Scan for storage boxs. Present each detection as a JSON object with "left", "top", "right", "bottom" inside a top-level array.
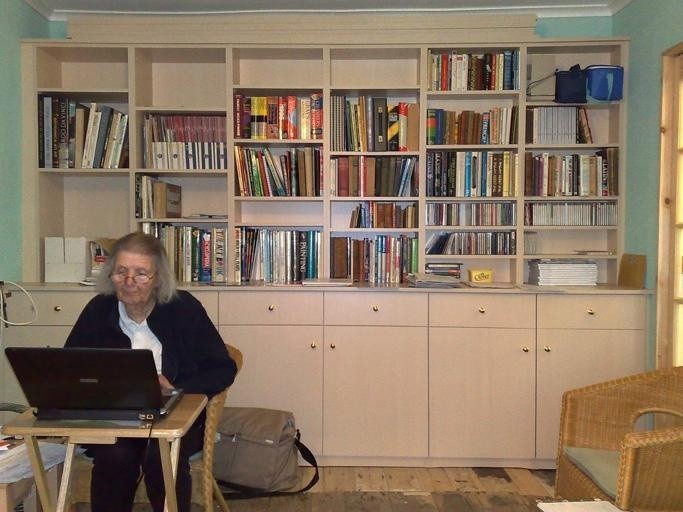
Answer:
[
  {"left": 44, "top": 261, "right": 91, "bottom": 283},
  {"left": 584, "top": 63, "right": 623, "bottom": 103},
  {"left": 552, "top": 63, "right": 588, "bottom": 103},
  {"left": 44, "top": 235, "right": 65, "bottom": 262},
  {"left": 152, "top": 181, "right": 183, "bottom": 218},
  {"left": 64, "top": 237, "right": 93, "bottom": 263}
]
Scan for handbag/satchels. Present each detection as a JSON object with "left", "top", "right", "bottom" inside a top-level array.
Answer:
[
  {"left": 589, "top": 65, "right": 624, "bottom": 101},
  {"left": 555, "top": 65, "right": 588, "bottom": 102}
]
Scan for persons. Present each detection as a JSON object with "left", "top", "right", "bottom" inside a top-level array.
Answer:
[{"left": 63, "top": 229, "right": 238, "bottom": 512}]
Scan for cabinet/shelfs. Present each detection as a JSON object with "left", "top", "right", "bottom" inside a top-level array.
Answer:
[
  {"left": 0, "top": 286, "right": 218, "bottom": 422},
  {"left": 427, "top": 292, "right": 650, "bottom": 470},
  {"left": 216, "top": 289, "right": 429, "bottom": 468},
  {"left": 18, "top": 38, "right": 632, "bottom": 289}
]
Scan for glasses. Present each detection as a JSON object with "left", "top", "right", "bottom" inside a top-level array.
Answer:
[{"left": 109, "top": 272, "right": 151, "bottom": 285}]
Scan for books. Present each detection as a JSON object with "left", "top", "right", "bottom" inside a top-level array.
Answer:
[
  {"left": 350, "top": 203, "right": 418, "bottom": 228},
  {"left": 328, "top": 157, "right": 420, "bottom": 198},
  {"left": 183, "top": 212, "right": 227, "bottom": 219},
  {"left": 425, "top": 230, "right": 515, "bottom": 254},
  {"left": 426, "top": 104, "right": 518, "bottom": 145},
  {"left": 427, "top": 51, "right": 519, "bottom": 91},
  {"left": 524, "top": 230, "right": 538, "bottom": 255},
  {"left": 141, "top": 112, "right": 227, "bottom": 169},
  {"left": 330, "top": 95, "right": 418, "bottom": 151},
  {"left": 525, "top": 107, "right": 596, "bottom": 145},
  {"left": 233, "top": 92, "right": 324, "bottom": 140},
  {"left": 234, "top": 227, "right": 324, "bottom": 285},
  {"left": 425, "top": 202, "right": 517, "bottom": 226},
  {"left": 38, "top": 92, "right": 129, "bottom": 168},
  {"left": 578, "top": 250, "right": 610, "bottom": 256},
  {"left": 524, "top": 149, "right": 619, "bottom": 196},
  {"left": 530, "top": 260, "right": 597, "bottom": 285},
  {"left": 330, "top": 234, "right": 416, "bottom": 283},
  {"left": 426, "top": 149, "right": 518, "bottom": 197},
  {"left": 141, "top": 221, "right": 227, "bottom": 285},
  {"left": 405, "top": 273, "right": 461, "bottom": 289},
  {"left": 464, "top": 282, "right": 514, "bottom": 289},
  {"left": 132, "top": 171, "right": 178, "bottom": 216},
  {"left": 425, "top": 261, "right": 462, "bottom": 287},
  {"left": 524, "top": 202, "right": 618, "bottom": 227},
  {"left": 233, "top": 144, "right": 324, "bottom": 197}
]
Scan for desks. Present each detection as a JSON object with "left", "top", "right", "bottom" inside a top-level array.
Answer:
[{"left": 0, "top": 391, "right": 208, "bottom": 512}]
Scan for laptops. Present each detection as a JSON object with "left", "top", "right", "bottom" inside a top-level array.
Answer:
[{"left": 4, "top": 346, "right": 184, "bottom": 423}]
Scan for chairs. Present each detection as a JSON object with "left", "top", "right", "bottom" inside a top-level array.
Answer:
[
  {"left": 55, "top": 341, "right": 244, "bottom": 512},
  {"left": 553, "top": 365, "right": 682, "bottom": 512}
]
